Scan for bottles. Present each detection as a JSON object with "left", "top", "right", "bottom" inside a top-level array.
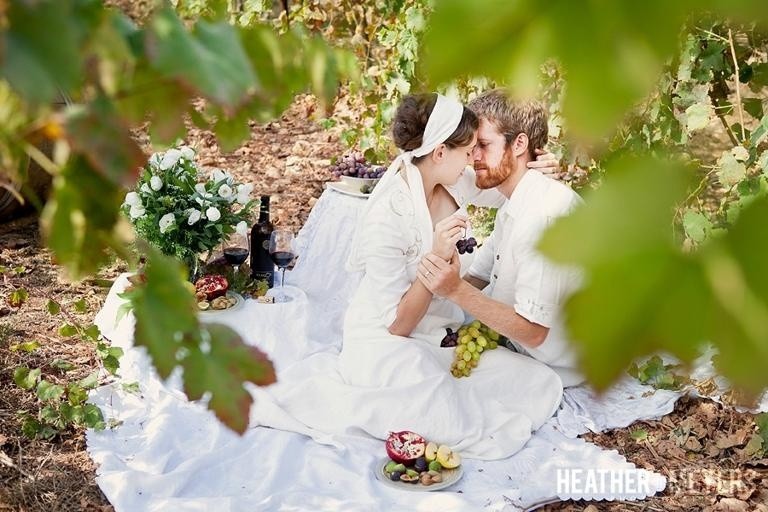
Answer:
[{"left": 250, "top": 195, "right": 273, "bottom": 290}]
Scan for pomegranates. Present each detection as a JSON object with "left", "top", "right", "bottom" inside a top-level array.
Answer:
[
  {"left": 386, "top": 431, "right": 426, "bottom": 466},
  {"left": 196, "top": 274, "right": 229, "bottom": 301}
]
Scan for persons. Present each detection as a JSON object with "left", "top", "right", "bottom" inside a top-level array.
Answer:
[
  {"left": 245, "top": 90, "right": 564, "bottom": 460},
  {"left": 414, "top": 86, "right": 587, "bottom": 388}
]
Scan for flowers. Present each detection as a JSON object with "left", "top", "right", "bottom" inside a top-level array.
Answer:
[{"left": 125, "top": 149, "right": 270, "bottom": 262}]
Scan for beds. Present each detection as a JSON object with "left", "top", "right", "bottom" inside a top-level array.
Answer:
[{"left": 276, "top": 189, "right": 367, "bottom": 285}]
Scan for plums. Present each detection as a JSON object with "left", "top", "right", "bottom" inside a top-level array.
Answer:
[
  {"left": 414, "top": 457, "right": 428, "bottom": 473},
  {"left": 428, "top": 460, "right": 442, "bottom": 472}
]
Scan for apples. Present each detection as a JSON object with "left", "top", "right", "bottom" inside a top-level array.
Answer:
[{"left": 425, "top": 442, "right": 461, "bottom": 469}]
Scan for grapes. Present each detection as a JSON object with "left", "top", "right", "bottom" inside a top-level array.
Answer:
[
  {"left": 328, "top": 155, "right": 388, "bottom": 193},
  {"left": 440, "top": 319, "right": 499, "bottom": 378},
  {"left": 456, "top": 237, "right": 477, "bottom": 254},
  {"left": 391, "top": 471, "right": 400, "bottom": 481}
]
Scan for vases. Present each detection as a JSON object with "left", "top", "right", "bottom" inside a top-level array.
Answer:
[{"left": 183, "top": 249, "right": 199, "bottom": 285}]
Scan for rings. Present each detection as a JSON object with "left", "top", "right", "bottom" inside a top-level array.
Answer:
[{"left": 425, "top": 271, "right": 430, "bottom": 278}]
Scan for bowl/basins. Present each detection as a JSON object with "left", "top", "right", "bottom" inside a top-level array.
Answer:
[{"left": 341, "top": 165, "right": 388, "bottom": 192}]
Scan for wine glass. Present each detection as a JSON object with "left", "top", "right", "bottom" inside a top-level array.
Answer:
[
  {"left": 221, "top": 232, "right": 249, "bottom": 280},
  {"left": 269, "top": 230, "right": 299, "bottom": 301}
]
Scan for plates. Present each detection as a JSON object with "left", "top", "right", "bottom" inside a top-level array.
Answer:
[
  {"left": 326, "top": 181, "right": 372, "bottom": 197},
  {"left": 195, "top": 290, "right": 245, "bottom": 315},
  {"left": 375, "top": 455, "right": 464, "bottom": 492}
]
